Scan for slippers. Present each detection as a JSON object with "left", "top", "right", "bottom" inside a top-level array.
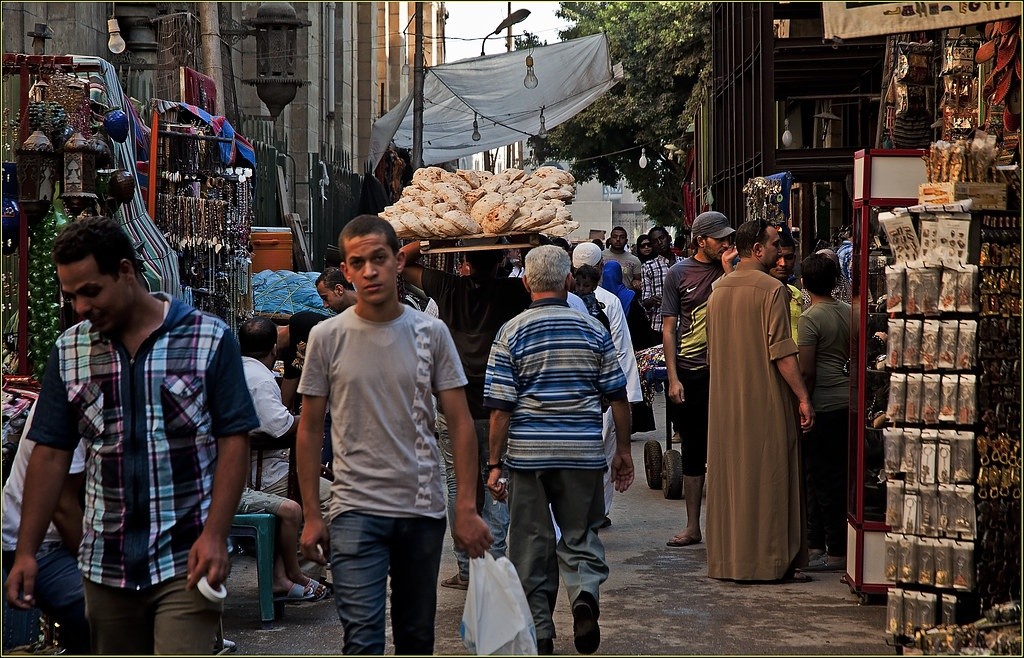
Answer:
[
  {"left": 304, "top": 578, "right": 328, "bottom": 603},
  {"left": 781, "top": 570, "right": 813, "bottom": 584},
  {"left": 274, "top": 583, "right": 315, "bottom": 603},
  {"left": 666, "top": 532, "right": 700, "bottom": 547}
]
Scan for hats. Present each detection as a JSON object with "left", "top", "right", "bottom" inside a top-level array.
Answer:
[
  {"left": 692, "top": 211, "right": 737, "bottom": 239},
  {"left": 571, "top": 241, "right": 602, "bottom": 269}
]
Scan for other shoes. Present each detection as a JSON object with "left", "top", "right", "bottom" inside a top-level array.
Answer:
[
  {"left": 798, "top": 555, "right": 847, "bottom": 573},
  {"left": 536, "top": 639, "right": 553, "bottom": 655},
  {"left": 571, "top": 597, "right": 601, "bottom": 655}
]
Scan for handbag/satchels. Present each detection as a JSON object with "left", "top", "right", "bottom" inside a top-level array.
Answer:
[{"left": 459, "top": 552, "right": 539, "bottom": 655}]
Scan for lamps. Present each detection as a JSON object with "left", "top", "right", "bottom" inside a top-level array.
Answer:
[{"left": 812, "top": 101, "right": 859, "bottom": 141}]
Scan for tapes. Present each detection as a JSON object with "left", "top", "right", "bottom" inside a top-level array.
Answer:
[{"left": 197, "top": 576, "right": 227, "bottom": 603}]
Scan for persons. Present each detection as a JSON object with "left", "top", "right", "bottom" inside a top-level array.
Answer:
[
  {"left": 482, "top": 234, "right": 635, "bottom": 655},
  {"left": 398, "top": 241, "right": 533, "bottom": 590},
  {"left": 316, "top": 267, "right": 358, "bottom": 315},
  {"left": 2, "top": 399, "right": 87, "bottom": 655},
  {"left": 296, "top": 215, "right": 494, "bottom": 655},
  {"left": 7, "top": 217, "right": 260, "bottom": 655},
  {"left": 276, "top": 311, "right": 329, "bottom": 409},
  {"left": 660, "top": 211, "right": 738, "bottom": 547},
  {"left": 707, "top": 218, "right": 851, "bottom": 580},
  {"left": 601, "top": 224, "right": 686, "bottom": 353},
  {"left": 573, "top": 242, "right": 644, "bottom": 527},
  {"left": 238, "top": 316, "right": 334, "bottom": 602}
]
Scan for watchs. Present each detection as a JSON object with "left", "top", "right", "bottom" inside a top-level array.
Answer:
[{"left": 487, "top": 461, "right": 502, "bottom": 470}]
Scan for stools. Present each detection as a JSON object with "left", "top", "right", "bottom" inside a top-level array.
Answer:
[{"left": 226, "top": 513, "right": 284, "bottom": 620}]
